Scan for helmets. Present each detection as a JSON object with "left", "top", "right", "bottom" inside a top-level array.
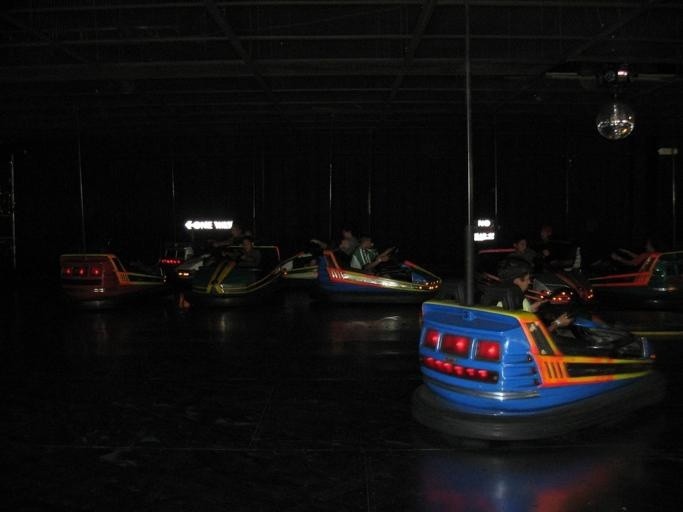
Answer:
[{"left": 504, "top": 258, "right": 535, "bottom": 272}]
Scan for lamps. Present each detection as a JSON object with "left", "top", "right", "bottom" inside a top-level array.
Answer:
[{"left": 596, "top": 34, "right": 636, "bottom": 140}]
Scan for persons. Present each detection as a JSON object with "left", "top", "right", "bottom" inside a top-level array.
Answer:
[
  {"left": 340, "top": 228, "right": 391, "bottom": 269},
  {"left": 231, "top": 224, "right": 254, "bottom": 261},
  {"left": 497, "top": 225, "right": 574, "bottom": 332}
]
